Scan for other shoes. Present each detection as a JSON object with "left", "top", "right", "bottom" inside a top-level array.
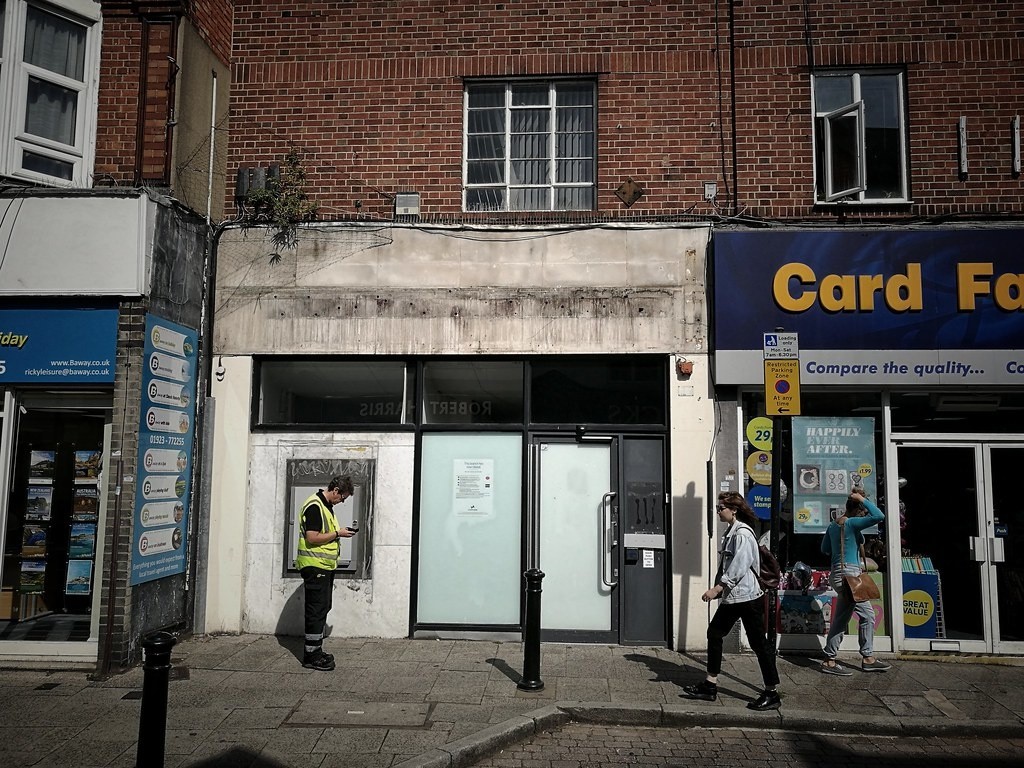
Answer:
[
  {"left": 821, "top": 662, "right": 852, "bottom": 675},
  {"left": 682, "top": 681, "right": 717, "bottom": 701},
  {"left": 322, "top": 651, "right": 334, "bottom": 660},
  {"left": 747, "top": 689, "right": 781, "bottom": 711},
  {"left": 862, "top": 658, "right": 892, "bottom": 671},
  {"left": 302, "top": 658, "right": 335, "bottom": 670}
]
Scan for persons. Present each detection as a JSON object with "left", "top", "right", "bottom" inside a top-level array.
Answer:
[
  {"left": 683, "top": 491, "right": 782, "bottom": 711},
  {"left": 821, "top": 486, "right": 891, "bottom": 676},
  {"left": 296, "top": 475, "right": 356, "bottom": 670}
]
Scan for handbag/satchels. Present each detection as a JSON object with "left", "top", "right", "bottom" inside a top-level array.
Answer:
[{"left": 842, "top": 572, "right": 880, "bottom": 602}]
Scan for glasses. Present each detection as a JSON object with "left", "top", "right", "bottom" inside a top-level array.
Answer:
[{"left": 716, "top": 505, "right": 737, "bottom": 510}]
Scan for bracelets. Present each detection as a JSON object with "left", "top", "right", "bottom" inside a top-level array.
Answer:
[{"left": 335, "top": 531, "right": 338, "bottom": 538}]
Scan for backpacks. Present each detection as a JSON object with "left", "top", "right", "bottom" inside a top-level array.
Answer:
[{"left": 736, "top": 527, "right": 780, "bottom": 590}]
